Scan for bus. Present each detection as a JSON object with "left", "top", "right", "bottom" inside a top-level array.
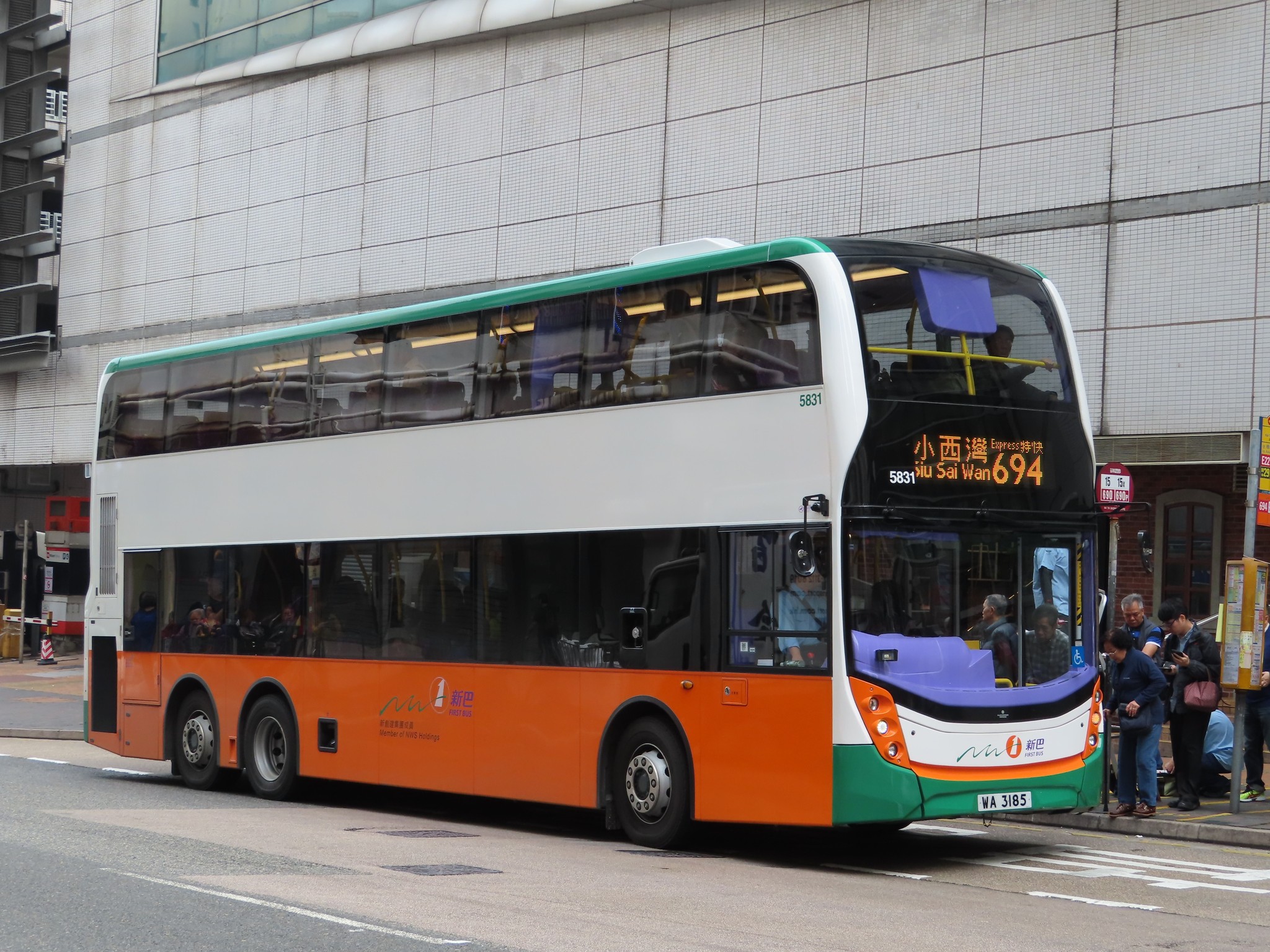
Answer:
[
  {"left": 80, "top": 232, "right": 1155, "bottom": 836},
  {"left": 41, "top": 496, "right": 92, "bottom": 634}
]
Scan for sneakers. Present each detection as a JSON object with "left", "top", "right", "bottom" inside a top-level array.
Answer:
[
  {"left": 1133, "top": 801, "right": 1155, "bottom": 816},
  {"left": 1239, "top": 785, "right": 1266, "bottom": 802},
  {"left": 1109, "top": 802, "right": 1136, "bottom": 817}
]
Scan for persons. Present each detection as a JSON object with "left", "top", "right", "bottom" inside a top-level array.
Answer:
[
  {"left": 180, "top": 570, "right": 223, "bottom": 652},
  {"left": 982, "top": 594, "right": 1018, "bottom": 689},
  {"left": 662, "top": 289, "right": 691, "bottom": 318},
  {"left": 1118, "top": 593, "right": 1163, "bottom": 770},
  {"left": 1234, "top": 602, "right": 1270, "bottom": 802},
  {"left": 131, "top": 592, "right": 156, "bottom": 653},
  {"left": 297, "top": 588, "right": 342, "bottom": 659},
  {"left": 352, "top": 378, "right": 376, "bottom": 430},
  {"left": 1025, "top": 605, "right": 1068, "bottom": 686},
  {"left": 271, "top": 604, "right": 295, "bottom": 657},
  {"left": 365, "top": 338, "right": 427, "bottom": 412},
  {"left": 1032, "top": 547, "right": 1068, "bottom": 633},
  {"left": 972, "top": 325, "right": 1057, "bottom": 402},
  {"left": 1157, "top": 596, "right": 1222, "bottom": 810},
  {"left": 172, "top": 609, "right": 205, "bottom": 647},
  {"left": 1101, "top": 626, "right": 1167, "bottom": 817},
  {"left": 770, "top": 563, "right": 827, "bottom": 669},
  {"left": 390, "top": 574, "right": 419, "bottom": 628},
  {"left": 1165, "top": 709, "right": 1244, "bottom": 799}
]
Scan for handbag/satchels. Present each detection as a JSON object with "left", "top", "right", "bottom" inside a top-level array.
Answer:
[
  {"left": 1118, "top": 703, "right": 1152, "bottom": 734},
  {"left": 1183, "top": 669, "right": 1220, "bottom": 707}
]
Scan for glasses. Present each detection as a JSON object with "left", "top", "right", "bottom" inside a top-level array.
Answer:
[
  {"left": 1161, "top": 618, "right": 1178, "bottom": 627},
  {"left": 1102, "top": 647, "right": 1119, "bottom": 657}
]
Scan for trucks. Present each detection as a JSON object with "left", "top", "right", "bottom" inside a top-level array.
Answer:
[{"left": 626, "top": 553, "right": 706, "bottom": 672}]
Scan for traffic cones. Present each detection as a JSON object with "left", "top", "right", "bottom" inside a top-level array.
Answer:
[{"left": 38, "top": 634, "right": 58, "bottom": 666}]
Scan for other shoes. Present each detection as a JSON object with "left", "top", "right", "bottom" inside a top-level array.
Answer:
[
  {"left": 1205, "top": 779, "right": 1231, "bottom": 797},
  {"left": 1167, "top": 799, "right": 1182, "bottom": 808},
  {"left": 1178, "top": 799, "right": 1200, "bottom": 810}
]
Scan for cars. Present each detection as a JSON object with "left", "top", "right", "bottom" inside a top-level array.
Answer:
[{"left": 1162, "top": 612, "right": 1220, "bottom": 654}]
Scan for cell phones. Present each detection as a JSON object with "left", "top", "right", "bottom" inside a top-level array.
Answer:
[{"left": 1172, "top": 649, "right": 1184, "bottom": 660}]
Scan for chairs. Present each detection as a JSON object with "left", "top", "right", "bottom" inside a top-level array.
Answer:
[{"left": 121, "top": 372, "right": 534, "bottom": 457}]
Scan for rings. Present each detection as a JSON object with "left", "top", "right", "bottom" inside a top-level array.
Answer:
[{"left": 1132, "top": 708, "right": 1134, "bottom": 710}]
